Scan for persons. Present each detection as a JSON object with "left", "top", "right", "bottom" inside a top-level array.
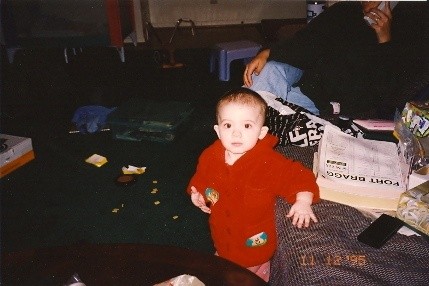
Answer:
[
  {"left": 240, "top": 0, "right": 429, "bottom": 115},
  {"left": 187, "top": 86, "right": 321, "bottom": 283}
]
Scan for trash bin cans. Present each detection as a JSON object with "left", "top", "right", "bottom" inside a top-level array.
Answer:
[{"left": 306, "top": 0, "right": 326, "bottom": 24}]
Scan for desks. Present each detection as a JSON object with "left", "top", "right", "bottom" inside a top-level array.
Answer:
[{"left": 0, "top": 244, "right": 269, "bottom": 286}]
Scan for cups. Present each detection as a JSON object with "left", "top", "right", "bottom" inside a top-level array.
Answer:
[{"left": 306, "top": 0, "right": 325, "bottom": 24}]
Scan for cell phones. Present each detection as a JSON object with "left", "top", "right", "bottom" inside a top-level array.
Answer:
[
  {"left": 364, "top": 1, "right": 399, "bottom": 28},
  {"left": 357, "top": 213, "right": 404, "bottom": 248}
]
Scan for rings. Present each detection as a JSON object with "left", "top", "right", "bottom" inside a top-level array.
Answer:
[{"left": 375, "top": 16, "right": 381, "bottom": 24}]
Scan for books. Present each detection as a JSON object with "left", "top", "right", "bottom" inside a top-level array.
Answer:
[{"left": 312, "top": 124, "right": 405, "bottom": 209}]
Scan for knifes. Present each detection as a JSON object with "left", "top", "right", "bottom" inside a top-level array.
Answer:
[{"left": 106, "top": 116, "right": 172, "bottom": 126}]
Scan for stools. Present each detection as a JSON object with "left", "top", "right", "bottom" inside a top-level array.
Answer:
[{"left": 209, "top": 40, "right": 262, "bottom": 82}]
